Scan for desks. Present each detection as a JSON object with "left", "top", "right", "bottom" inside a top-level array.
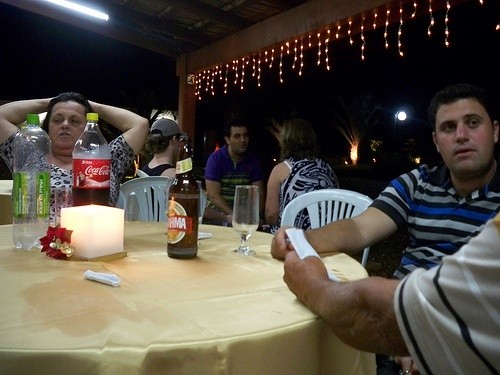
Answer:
[{"left": 0, "top": 221, "right": 377, "bottom": 375}]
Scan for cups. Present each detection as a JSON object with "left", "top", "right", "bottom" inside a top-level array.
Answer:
[
  {"left": 197, "top": 181, "right": 202, "bottom": 223},
  {"left": 55, "top": 187, "right": 73, "bottom": 226}
]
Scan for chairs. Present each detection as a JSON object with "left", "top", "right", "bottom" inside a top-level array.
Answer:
[
  {"left": 114, "top": 175, "right": 206, "bottom": 225},
  {"left": 279, "top": 188, "right": 376, "bottom": 269}
]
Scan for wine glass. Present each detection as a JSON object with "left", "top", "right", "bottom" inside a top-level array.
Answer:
[{"left": 232, "top": 185, "right": 260, "bottom": 257}]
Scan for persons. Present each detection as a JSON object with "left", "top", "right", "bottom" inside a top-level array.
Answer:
[
  {"left": 282, "top": 209, "right": 500, "bottom": 375},
  {"left": 133, "top": 117, "right": 189, "bottom": 222},
  {"left": 0, "top": 92, "right": 150, "bottom": 223},
  {"left": 264, "top": 119, "right": 340, "bottom": 234},
  {"left": 271, "top": 85, "right": 500, "bottom": 280},
  {"left": 203, "top": 122, "right": 266, "bottom": 228}
]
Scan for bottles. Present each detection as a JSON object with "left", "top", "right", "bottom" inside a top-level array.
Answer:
[
  {"left": 167, "top": 134, "right": 200, "bottom": 260},
  {"left": 71, "top": 113, "right": 110, "bottom": 206},
  {"left": 12, "top": 113, "right": 51, "bottom": 251}
]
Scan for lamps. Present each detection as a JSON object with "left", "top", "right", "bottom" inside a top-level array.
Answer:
[{"left": 47, "top": 0, "right": 109, "bottom": 20}]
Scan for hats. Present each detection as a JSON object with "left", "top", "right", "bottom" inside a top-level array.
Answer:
[{"left": 150, "top": 118, "right": 188, "bottom": 137}]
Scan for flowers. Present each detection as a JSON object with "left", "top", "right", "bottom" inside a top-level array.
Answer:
[{"left": 39, "top": 223, "right": 73, "bottom": 258}]
